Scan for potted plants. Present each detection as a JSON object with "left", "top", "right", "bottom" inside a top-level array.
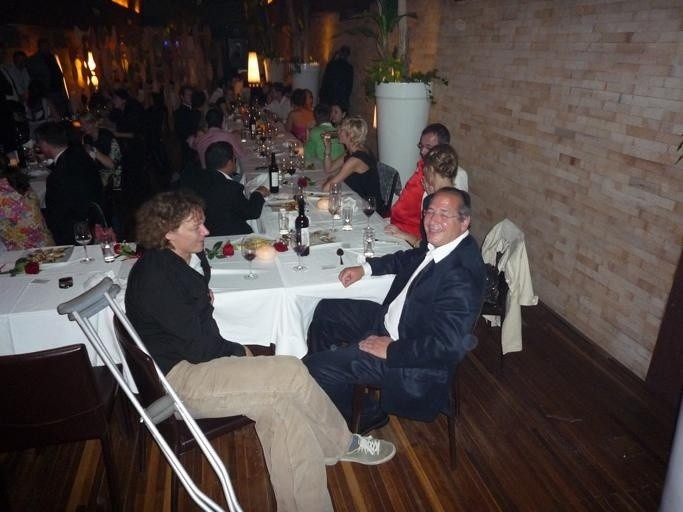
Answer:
[
  {"left": 290, "top": 56, "right": 321, "bottom": 101},
  {"left": 262, "top": 40, "right": 285, "bottom": 82},
  {"left": 334, "top": 1, "right": 451, "bottom": 205}
]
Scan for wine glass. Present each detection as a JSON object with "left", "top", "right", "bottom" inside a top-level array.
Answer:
[
  {"left": 279, "top": 140, "right": 304, "bottom": 184},
  {"left": 319, "top": 130, "right": 339, "bottom": 139},
  {"left": 240, "top": 237, "right": 259, "bottom": 281},
  {"left": 362, "top": 198, "right": 377, "bottom": 230},
  {"left": 327, "top": 184, "right": 342, "bottom": 233},
  {"left": 72, "top": 220, "right": 95, "bottom": 263},
  {"left": 289, "top": 232, "right": 309, "bottom": 272},
  {"left": 230, "top": 93, "right": 279, "bottom": 158}
]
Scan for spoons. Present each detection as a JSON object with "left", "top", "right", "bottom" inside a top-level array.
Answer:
[{"left": 336, "top": 248, "right": 345, "bottom": 265}]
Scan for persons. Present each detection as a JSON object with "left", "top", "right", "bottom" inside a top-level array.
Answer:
[
  {"left": 301, "top": 187, "right": 486, "bottom": 437},
  {"left": 125, "top": 188, "right": 396, "bottom": 512}
]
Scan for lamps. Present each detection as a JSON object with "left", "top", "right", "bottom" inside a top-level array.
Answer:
[{"left": 246, "top": 49, "right": 260, "bottom": 89}]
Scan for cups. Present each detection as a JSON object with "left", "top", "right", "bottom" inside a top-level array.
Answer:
[
  {"left": 99, "top": 237, "right": 115, "bottom": 265},
  {"left": 341, "top": 206, "right": 353, "bottom": 232},
  {"left": 279, "top": 207, "right": 289, "bottom": 234},
  {"left": 290, "top": 182, "right": 304, "bottom": 199},
  {"left": 363, "top": 232, "right": 376, "bottom": 256}
]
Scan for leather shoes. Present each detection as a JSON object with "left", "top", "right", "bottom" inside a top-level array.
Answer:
[{"left": 359, "top": 405, "right": 389, "bottom": 435}]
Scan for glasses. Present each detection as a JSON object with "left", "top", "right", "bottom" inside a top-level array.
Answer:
[{"left": 417, "top": 144, "right": 432, "bottom": 151}]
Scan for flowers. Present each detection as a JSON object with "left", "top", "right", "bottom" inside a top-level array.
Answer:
[
  {"left": 109, "top": 241, "right": 143, "bottom": 259},
  {"left": 296, "top": 176, "right": 316, "bottom": 188},
  {"left": 0, "top": 255, "right": 39, "bottom": 277},
  {"left": 203, "top": 237, "right": 234, "bottom": 259},
  {"left": 271, "top": 227, "right": 294, "bottom": 252}
]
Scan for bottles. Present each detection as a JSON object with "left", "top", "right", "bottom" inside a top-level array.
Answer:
[
  {"left": 293, "top": 198, "right": 311, "bottom": 257},
  {"left": 268, "top": 152, "right": 279, "bottom": 194}
]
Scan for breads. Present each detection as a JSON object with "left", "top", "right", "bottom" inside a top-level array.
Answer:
[
  {"left": 294, "top": 147, "right": 304, "bottom": 155},
  {"left": 316, "top": 198, "right": 329, "bottom": 211},
  {"left": 256, "top": 246, "right": 275, "bottom": 261}
]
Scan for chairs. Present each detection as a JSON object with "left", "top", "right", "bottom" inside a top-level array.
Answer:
[
  {"left": 111, "top": 302, "right": 268, "bottom": 512},
  {"left": 466, "top": 216, "right": 532, "bottom": 378},
  {"left": 347, "top": 274, "right": 486, "bottom": 472},
  {"left": 54, "top": 175, "right": 117, "bottom": 246},
  {"left": 0, "top": 339, "right": 139, "bottom": 512}
]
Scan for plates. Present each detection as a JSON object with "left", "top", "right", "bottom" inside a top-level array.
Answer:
[{"left": 20, "top": 242, "right": 73, "bottom": 264}]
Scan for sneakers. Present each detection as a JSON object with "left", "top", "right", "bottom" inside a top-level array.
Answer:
[{"left": 338, "top": 433, "right": 396, "bottom": 465}]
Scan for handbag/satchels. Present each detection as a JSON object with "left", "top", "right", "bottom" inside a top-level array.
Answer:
[{"left": 484, "top": 264, "right": 500, "bottom": 306}]
